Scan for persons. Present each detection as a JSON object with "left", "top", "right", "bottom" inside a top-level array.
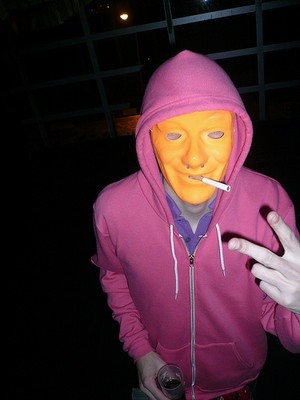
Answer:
[{"left": 93, "top": 50, "right": 300, "bottom": 400}]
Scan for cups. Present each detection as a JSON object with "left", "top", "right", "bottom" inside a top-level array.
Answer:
[{"left": 157, "top": 364, "right": 185, "bottom": 400}]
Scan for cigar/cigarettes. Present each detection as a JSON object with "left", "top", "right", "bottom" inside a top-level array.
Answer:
[{"left": 193, "top": 175, "right": 230, "bottom": 192}]
